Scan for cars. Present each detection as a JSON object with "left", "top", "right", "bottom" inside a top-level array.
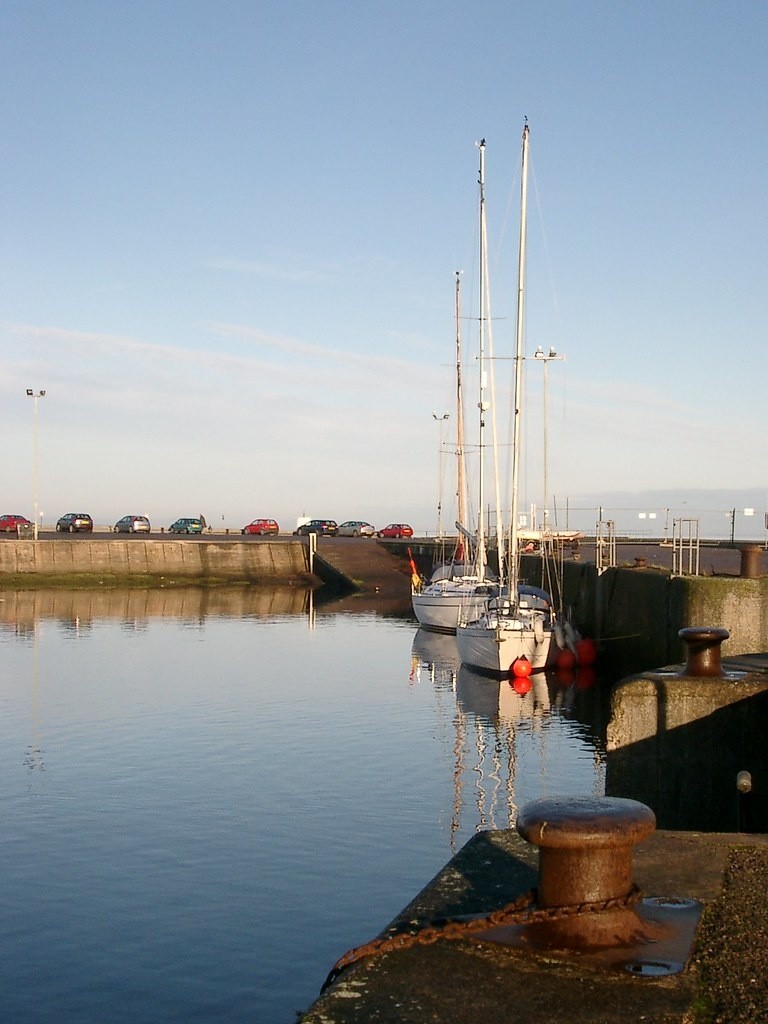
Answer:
[
  {"left": 0, "top": 515, "right": 31, "bottom": 534},
  {"left": 297, "top": 519, "right": 339, "bottom": 538},
  {"left": 113, "top": 515, "right": 151, "bottom": 533},
  {"left": 337, "top": 521, "right": 375, "bottom": 538},
  {"left": 168, "top": 518, "right": 202, "bottom": 534},
  {"left": 377, "top": 524, "right": 414, "bottom": 537},
  {"left": 242, "top": 519, "right": 279, "bottom": 535},
  {"left": 56, "top": 513, "right": 94, "bottom": 533}
]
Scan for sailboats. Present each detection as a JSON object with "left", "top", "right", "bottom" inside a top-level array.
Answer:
[
  {"left": 410, "top": 269, "right": 504, "bottom": 637},
  {"left": 452, "top": 664, "right": 556, "bottom": 840},
  {"left": 453, "top": 116, "right": 592, "bottom": 684}
]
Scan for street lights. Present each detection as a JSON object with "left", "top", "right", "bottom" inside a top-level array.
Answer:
[
  {"left": 434, "top": 411, "right": 450, "bottom": 537},
  {"left": 26, "top": 387, "right": 46, "bottom": 537}
]
